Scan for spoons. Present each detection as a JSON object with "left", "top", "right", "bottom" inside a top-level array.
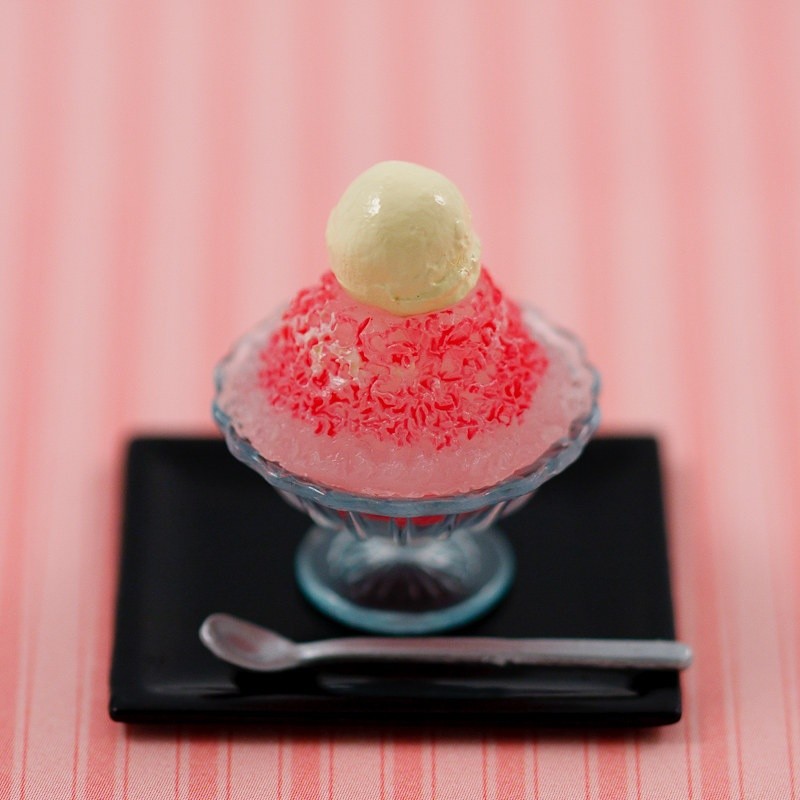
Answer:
[{"left": 202, "top": 613, "right": 694, "bottom": 674}]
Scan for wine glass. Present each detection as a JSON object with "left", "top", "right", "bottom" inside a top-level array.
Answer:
[{"left": 213, "top": 304, "right": 601, "bottom": 635}]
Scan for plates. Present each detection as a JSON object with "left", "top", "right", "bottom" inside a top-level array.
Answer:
[{"left": 110, "top": 435, "right": 682, "bottom": 732}]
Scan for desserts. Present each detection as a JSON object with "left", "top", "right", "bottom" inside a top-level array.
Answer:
[{"left": 229, "top": 149, "right": 586, "bottom": 527}]
